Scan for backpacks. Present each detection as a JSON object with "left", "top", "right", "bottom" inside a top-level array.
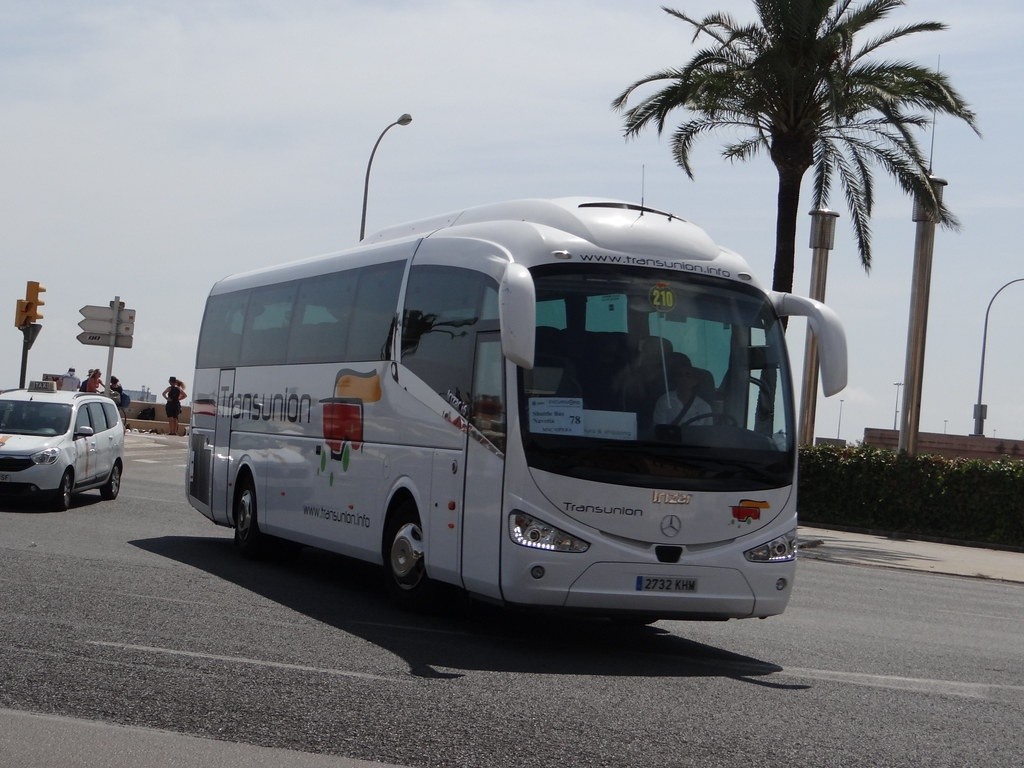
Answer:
[{"left": 120, "top": 391, "right": 130, "bottom": 407}]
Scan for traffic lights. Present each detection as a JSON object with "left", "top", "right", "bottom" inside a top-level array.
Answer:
[
  {"left": 26, "top": 281, "right": 46, "bottom": 323},
  {"left": 15, "top": 299, "right": 34, "bottom": 327}
]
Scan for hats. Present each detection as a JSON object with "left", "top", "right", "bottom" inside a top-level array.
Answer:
[
  {"left": 86, "top": 369, "right": 94, "bottom": 376},
  {"left": 168, "top": 377, "right": 177, "bottom": 382},
  {"left": 68, "top": 367, "right": 74, "bottom": 374}
]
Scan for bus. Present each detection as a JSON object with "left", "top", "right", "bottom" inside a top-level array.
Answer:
[{"left": 184, "top": 196, "right": 847, "bottom": 619}]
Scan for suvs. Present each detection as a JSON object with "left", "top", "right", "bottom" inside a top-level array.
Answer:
[{"left": 0, "top": 389, "right": 124, "bottom": 511}]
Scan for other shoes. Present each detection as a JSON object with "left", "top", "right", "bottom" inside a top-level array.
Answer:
[{"left": 169, "top": 432, "right": 176, "bottom": 436}]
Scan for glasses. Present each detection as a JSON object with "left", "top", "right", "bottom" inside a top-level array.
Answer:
[{"left": 96, "top": 372, "right": 103, "bottom": 375}]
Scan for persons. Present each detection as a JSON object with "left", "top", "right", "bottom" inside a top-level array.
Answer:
[
  {"left": 110, "top": 376, "right": 122, "bottom": 408},
  {"left": 86, "top": 369, "right": 105, "bottom": 393},
  {"left": 79, "top": 369, "right": 94, "bottom": 392},
  {"left": 163, "top": 377, "right": 187, "bottom": 435},
  {"left": 654, "top": 372, "right": 713, "bottom": 425},
  {"left": 59, "top": 368, "right": 80, "bottom": 391}
]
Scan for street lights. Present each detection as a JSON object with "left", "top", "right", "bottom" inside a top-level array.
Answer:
[
  {"left": 944, "top": 420, "right": 948, "bottom": 434},
  {"left": 359, "top": 114, "right": 412, "bottom": 239},
  {"left": 893, "top": 382, "right": 903, "bottom": 430},
  {"left": 993, "top": 429, "right": 996, "bottom": 438},
  {"left": 837, "top": 400, "right": 843, "bottom": 438}
]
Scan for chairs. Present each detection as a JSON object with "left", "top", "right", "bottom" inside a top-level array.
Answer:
[{"left": 222, "top": 322, "right": 718, "bottom": 421}]
